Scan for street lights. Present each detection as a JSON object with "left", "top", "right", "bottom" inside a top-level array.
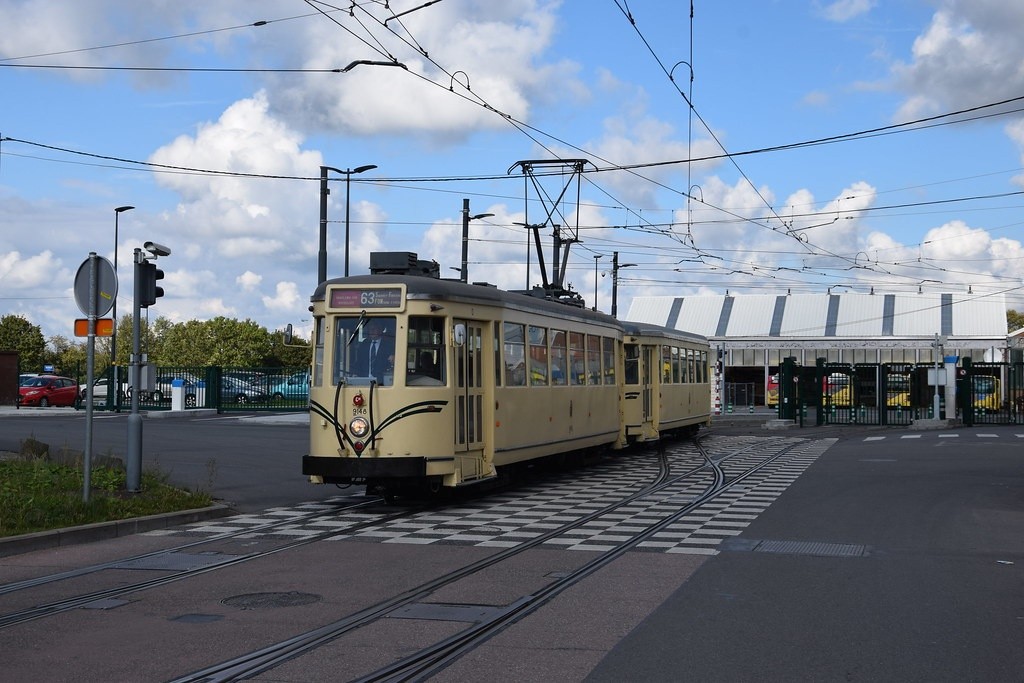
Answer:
[
  {"left": 318, "top": 164, "right": 376, "bottom": 276},
  {"left": 461, "top": 198, "right": 495, "bottom": 283},
  {"left": 609, "top": 251, "right": 639, "bottom": 321},
  {"left": 107, "top": 207, "right": 137, "bottom": 409}
]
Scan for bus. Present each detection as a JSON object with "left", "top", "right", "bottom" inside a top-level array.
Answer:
[
  {"left": 282, "top": 158, "right": 714, "bottom": 491},
  {"left": 972, "top": 375, "right": 1002, "bottom": 412},
  {"left": 887, "top": 374, "right": 911, "bottom": 409},
  {"left": 767, "top": 372, "right": 781, "bottom": 408},
  {"left": 822, "top": 373, "right": 854, "bottom": 409}
]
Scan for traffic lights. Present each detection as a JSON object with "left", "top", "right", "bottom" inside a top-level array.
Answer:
[{"left": 143, "top": 264, "right": 165, "bottom": 308}]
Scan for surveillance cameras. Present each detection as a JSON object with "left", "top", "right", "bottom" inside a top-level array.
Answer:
[
  {"left": 143, "top": 240, "right": 171, "bottom": 257},
  {"left": 601, "top": 271, "right": 606, "bottom": 277}
]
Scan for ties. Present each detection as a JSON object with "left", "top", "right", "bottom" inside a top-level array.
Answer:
[{"left": 370, "top": 342, "right": 377, "bottom": 377}]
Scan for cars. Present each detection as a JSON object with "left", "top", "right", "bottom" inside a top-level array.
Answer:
[
  {"left": 80, "top": 371, "right": 312, "bottom": 408},
  {"left": 20, "top": 372, "right": 86, "bottom": 407}
]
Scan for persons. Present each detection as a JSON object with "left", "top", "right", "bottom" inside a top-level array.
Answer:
[
  {"left": 415, "top": 351, "right": 439, "bottom": 380},
  {"left": 351, "top": 318, "right": 395, "bottom": 385}
]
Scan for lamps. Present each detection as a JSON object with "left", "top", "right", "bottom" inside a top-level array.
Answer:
[
  {"left": 917, "top": 286, "right": 923, "bottom": 295},
  {"left": 966, "top": 285, "right": 974, "bottom": 294},
  {"left": 725, "top": 289, "right": 730, "bottom": 298},
  {"left": 786, "top": 289, "right": 792, "bottom": 297},
  {"left": 826, "top": 288, "right": 831, "bottom": 296},
  {"left": 869, "top": 286, "right": 875, "bottom": 295}
]
